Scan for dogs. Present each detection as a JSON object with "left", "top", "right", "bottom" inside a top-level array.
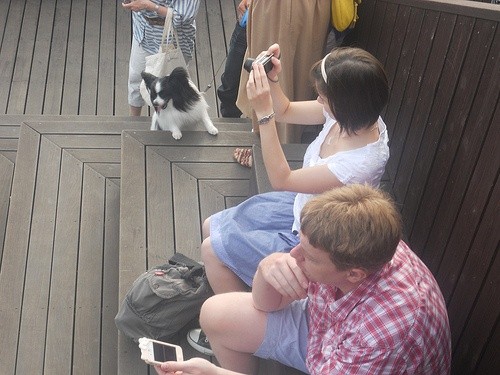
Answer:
[{"left": 140, "top": 66, "right": 219, "bottom": 140}]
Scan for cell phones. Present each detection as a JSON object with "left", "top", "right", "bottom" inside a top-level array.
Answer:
[{"left": 137, "top": 338, "right": 183, "bottom": 365}]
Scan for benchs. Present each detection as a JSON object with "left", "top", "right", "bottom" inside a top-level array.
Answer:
[{"left": 250, "top": 143, "right": 411, "bottom": 375}]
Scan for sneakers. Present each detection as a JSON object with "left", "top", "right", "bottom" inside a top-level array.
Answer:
[{"left": 186, "top": 328, "right": 214, "bottom": 355}]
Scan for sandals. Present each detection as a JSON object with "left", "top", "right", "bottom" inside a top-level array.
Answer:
[{"left": 233, "top": 148, "right": 254, "bottom": 168}]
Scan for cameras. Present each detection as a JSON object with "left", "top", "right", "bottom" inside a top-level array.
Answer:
[{"left": 244, "top": 53, "right": 274, "bottom": 74}]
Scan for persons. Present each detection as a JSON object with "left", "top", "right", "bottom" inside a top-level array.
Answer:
[
  {"left": 120, "top": 0, "right": 331, "bottom": 144},
  {"left": 186, "top": 44, "right": 391, "bottom": 357},
  {"left": 151, "top": 184, "right": 452, "bottom": 375}
]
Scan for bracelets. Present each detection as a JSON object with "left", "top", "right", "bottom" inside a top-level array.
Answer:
[{"left": 153, "top": 4, "right": 159, "bottom": 15}]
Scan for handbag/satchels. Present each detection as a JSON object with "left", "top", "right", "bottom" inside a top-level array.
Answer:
[
  {"left": 115, "top": 252, "right": 215, "bottom": 343},
  {"left": 140, "top": 7, "right": 191, "bottom": 108}
]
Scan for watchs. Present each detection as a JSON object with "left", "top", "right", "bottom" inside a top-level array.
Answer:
[{"left": 257, "top": 112, "right": 275, "bottom": 124}]
